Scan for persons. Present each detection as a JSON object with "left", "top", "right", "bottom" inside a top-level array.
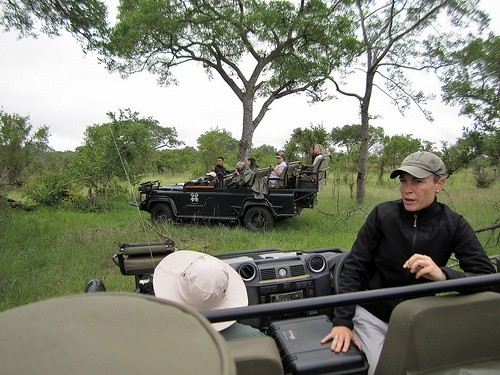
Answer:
[
  {"left": 197, "top": 143, "right": 324, "bottom": 189},
  {"left": 320, "top": 151, "right": 500, "bottom": 375}
]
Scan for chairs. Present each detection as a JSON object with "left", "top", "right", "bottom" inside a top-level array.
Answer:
[
  {"left": 264, "top": 161, "right": 300, "bottom": 189},
  {"left": 372, "top": 291, "right": 500, "bottom": 375},
  {"left": 225, "top": 336, "right": 284, "bottom": 375},
  {"left": 298, "top": 154, "right": 331, "bottom": 183}
]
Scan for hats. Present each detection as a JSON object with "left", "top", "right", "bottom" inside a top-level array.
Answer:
[
  {"left": 206, "top": 172, "right": 216, "bottom": 177},
  {"left": 390, "top": 151, "right": 445, "bottom": 180},
  {"left": 153, "top": 250, "right": 249, "bottom": 332}
]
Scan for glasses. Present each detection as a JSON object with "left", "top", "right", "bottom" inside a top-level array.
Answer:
[{"left": 275, "top": 157, "right": 282, "bottom": 159}]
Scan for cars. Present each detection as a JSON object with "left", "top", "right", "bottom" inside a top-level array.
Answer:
[{"left": 136, "top": 154, "right": 331, "bottom": 233}]
